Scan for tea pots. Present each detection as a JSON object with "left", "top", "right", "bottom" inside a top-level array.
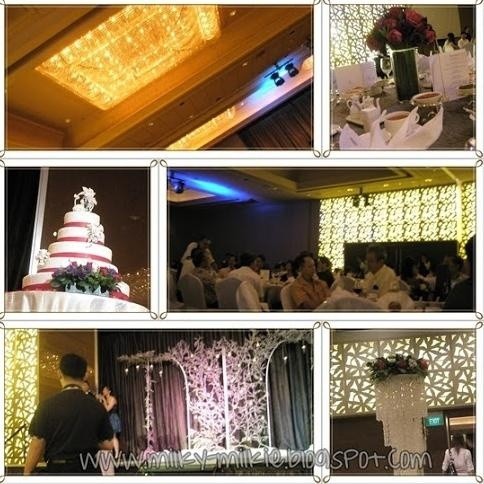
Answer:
[{"left": 347, "top": 94, "right": 381, "bottom": 123}]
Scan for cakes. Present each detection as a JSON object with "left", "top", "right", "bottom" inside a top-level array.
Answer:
[{"left": 22, "top": 211, "right": 131, "bottom": 302}]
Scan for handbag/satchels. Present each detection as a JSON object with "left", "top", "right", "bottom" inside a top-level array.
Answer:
[{"left": 445, "top": 448, "right": 458, "bottom": 475}]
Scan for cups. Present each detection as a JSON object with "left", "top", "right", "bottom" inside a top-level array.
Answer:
[
  {"left": 413, "top": 91, "right": 444, "bottom": 126},
  {"left": 383, "top": 110, "right": 420, "bottom": 135}
]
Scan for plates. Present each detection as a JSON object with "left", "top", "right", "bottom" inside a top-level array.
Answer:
[
  {"left": 331, "top": 123, "right": 340, "bottom": 136},
  {"left": 345, "top": 115, "right": 386, "bottom": 127}
]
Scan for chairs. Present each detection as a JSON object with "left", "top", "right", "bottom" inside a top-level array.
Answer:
[
  {"left": 168, "top": 269, "right": 414, "bottom": 310},
  {"left": 332, "top": 50, "right": 471, "bottom": 106}
]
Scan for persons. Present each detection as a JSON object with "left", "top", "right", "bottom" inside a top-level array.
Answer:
[
  {"left": 443, "top": 36, "right": 459, "bottom": 53},
  {"left": 440, "top": 432, "right": 475, "bottom": 475},
  {"left": 22, "top": 351, "right": 116, "bottom": 476},
  {"left": 82, "top": 379, "right": 96, "bottom": 399},
  {"left": 95, "top": 383, "right": 123, "bottom": 462},
  {"left": 458, "top": 31, "right": 469, "bottom": 48},
  {"left": 444, "top": 32, "right": 457, "bottom": 49}
]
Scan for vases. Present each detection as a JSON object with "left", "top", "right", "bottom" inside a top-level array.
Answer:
[{"left": 392, "top": 49, "right": 419, "bottom": 100}]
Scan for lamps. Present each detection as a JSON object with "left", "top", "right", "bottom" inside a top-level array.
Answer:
[
  {"left": 264, "top": 58, "right": 299, "bottom": 86},
  {"left": 167, "top": 169, "right": 186, "bottom": 194}
]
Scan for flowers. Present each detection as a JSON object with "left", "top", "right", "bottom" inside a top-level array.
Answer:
[
  {"left": 366, "top": 6, "right": 436, "bottom": 56},
  {"left": 365, "top": 352, "right": 432, "bottom": 383},
  {"left": 49, "top": 261, "right": 122, "bottom": 293}
]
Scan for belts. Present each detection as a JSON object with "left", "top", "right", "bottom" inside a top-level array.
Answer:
[{"left": 48, "top": 459, "right": 80, "bottom": 464}]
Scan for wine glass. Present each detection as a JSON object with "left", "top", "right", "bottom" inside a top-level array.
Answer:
[{"left": 380, "top": 58, "right": 392, "bottom": 80}]
